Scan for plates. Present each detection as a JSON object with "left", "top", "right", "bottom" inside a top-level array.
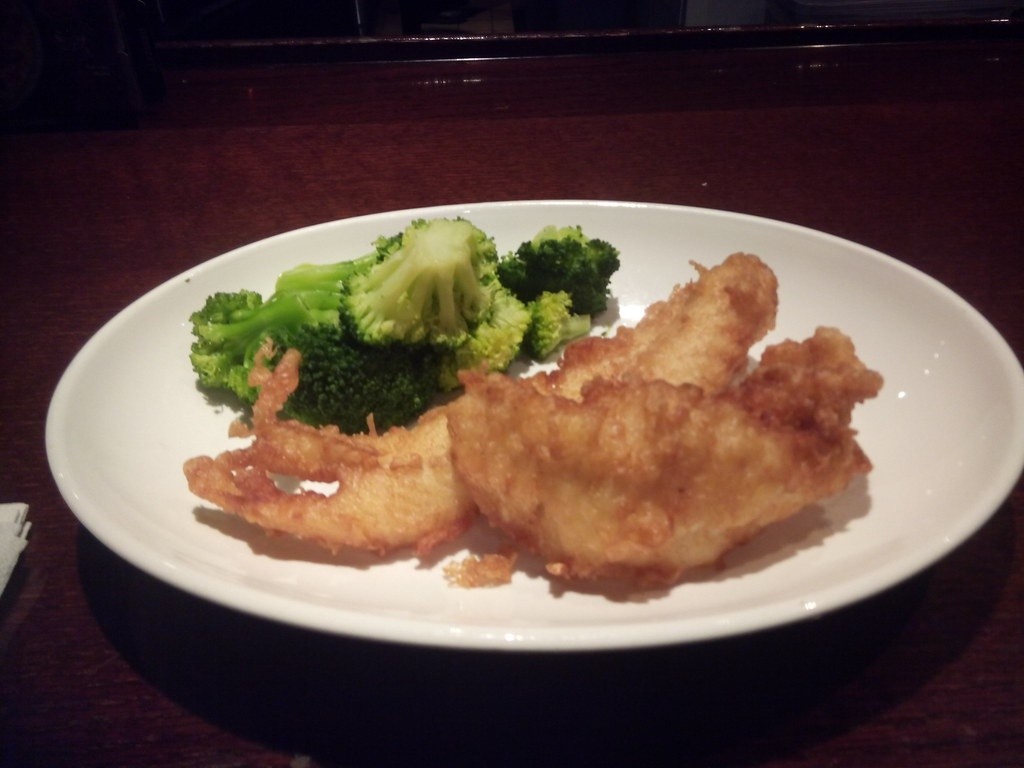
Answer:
[{"left": 44, "top": 202, "right": 1024, "bottom": 653}]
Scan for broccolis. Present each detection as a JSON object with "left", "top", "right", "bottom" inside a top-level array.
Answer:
[{"left": 190, "top": 216, "right": 621, "bottom": 434}]
